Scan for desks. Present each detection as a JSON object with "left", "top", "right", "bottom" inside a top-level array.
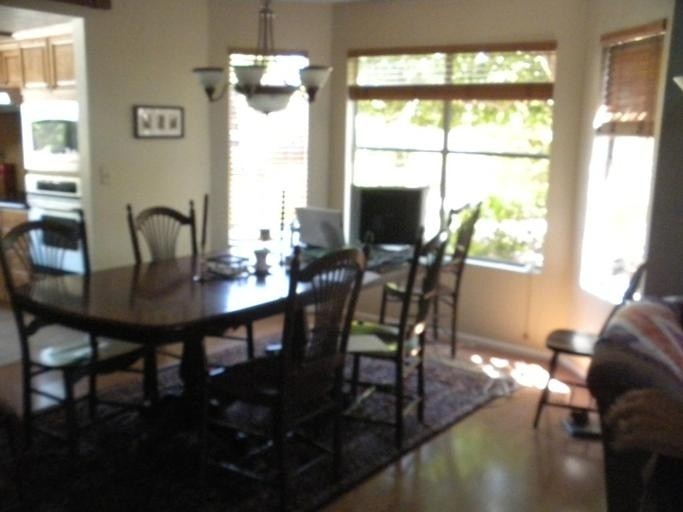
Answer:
[{"left": 12, "top": 231, "right": 418, "bottom": 512}]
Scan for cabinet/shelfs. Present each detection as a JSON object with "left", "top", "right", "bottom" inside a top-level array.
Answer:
[
  {"left": 0, "top": 33, "right": 74, "bottom": 90},
  {"left": 0, "top": 208, "right": 27, "bottom": 305}
]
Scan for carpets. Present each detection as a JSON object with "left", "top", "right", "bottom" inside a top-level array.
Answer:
[{"left": 0, "top": 324, "right": 524, "bottom": 512}]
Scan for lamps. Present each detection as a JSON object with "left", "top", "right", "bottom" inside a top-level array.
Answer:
[{"left": 191, "top": 0, "right": 334, "bottom": 116}]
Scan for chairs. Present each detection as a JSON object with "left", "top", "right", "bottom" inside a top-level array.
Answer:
[
  {"left": 380, "top": 200, "right": 485, "bottom": 357},
  {"left": 125, "top": 200, "right": 197, "bottom": 269},
  {"left": 199, "top": 239, "right": 371, "bottom": 512},
  {"left": 534, "top": 262, "right": 645, "bottom": 433},
  {"left": 340, "top": 229, "right": 451, "bottom": 431},
  {"left": 1, "top": 208, "right": 160, "bottom": 464}
]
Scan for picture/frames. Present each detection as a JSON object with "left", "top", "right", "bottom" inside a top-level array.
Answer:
[{"left": 133, "top": 104, "right": 184, "bottom": 138}]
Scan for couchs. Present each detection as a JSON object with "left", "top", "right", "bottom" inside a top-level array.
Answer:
[{"left": 587, "top": 294, "right": 682, "bottom": 512}]
